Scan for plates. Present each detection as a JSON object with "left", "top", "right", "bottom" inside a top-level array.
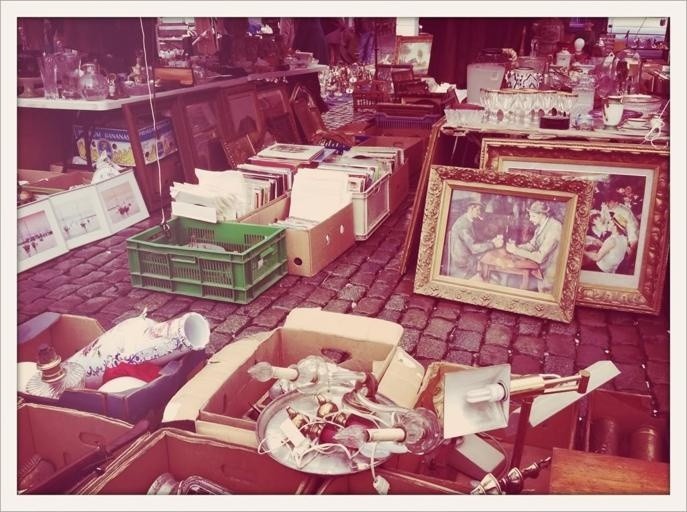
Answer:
[{"left": 621, "top": 125, "right": 652, "bottom": 131}]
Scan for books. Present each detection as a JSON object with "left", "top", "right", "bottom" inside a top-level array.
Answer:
[{"left": 170, "top": 141, "right": 407, "bottom": 232}]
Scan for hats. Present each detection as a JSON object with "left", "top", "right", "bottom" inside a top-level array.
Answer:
[
  {"left": 463, "top": 192, "right": 487, "bottom": 207},
  {"left": 526, "top": 200, "right": 550, "bottom": 213},
  {"left": 610, "top": 210, "right": 627, "bottom": 234}
]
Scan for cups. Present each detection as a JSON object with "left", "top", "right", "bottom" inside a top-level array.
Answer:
[
  {"left": 40, "top": 54, "right": 79, "bottom": 101},
  {"left": 627, "top": 118, "right": 649, "bottom": 126},
  {"left": 295, "top": 52, "right": 313, "bottom": 69},
  {"left": 601, "top": 102, "right": 622, "bottom": 131}
]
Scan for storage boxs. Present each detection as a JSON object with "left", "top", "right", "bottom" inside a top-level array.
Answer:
[
  {"left": 71, "top": 88, "right": 460, "bottom": 278},
  {"left": 17, "top": 306, "right": 670, "bottom": 495}
]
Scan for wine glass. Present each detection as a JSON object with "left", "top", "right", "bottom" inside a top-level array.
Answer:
[{"left": 479, "top": 87, "right": 576, "bottom": 131}]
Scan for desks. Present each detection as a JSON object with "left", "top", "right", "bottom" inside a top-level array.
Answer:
[{"left": 17, "top": 54, "right": 330, "bottom": 215}]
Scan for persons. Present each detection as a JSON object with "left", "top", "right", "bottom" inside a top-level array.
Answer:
[
  {"left": 507, "top": 200, "right": 566, "bottom": 290},
  {"left": 448, "top": 195, "right": 504, "bottom": 282},
  {"left": 606, "top": 193, "right": 639, "bottom": 251},
  {"left": 582, "top": 216, "right": 629, "bottom": 275},
  {"left": 216, "top": 17, "right": 378, "bottom": 70}
]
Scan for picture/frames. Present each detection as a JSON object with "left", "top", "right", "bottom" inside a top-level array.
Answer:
[
  {"left": 17, "top": 86, "right": 331, "bottom": 275},
  {"left": 391, "top": 35, "right": 436, "bottom": 80},
  {"left": 400, "top": 112, "right": 669, "bottom": 323}
]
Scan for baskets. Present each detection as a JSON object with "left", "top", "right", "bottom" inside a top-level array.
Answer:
[{"left": 126, "top": 215, "right": 288, "bottom": 305}]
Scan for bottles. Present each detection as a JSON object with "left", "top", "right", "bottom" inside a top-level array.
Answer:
[
  {"left": 53, "top": 312, "right": 210, "bottom": 386},
  {"left": 78, "top": 64, "right": 109, "bottom": 101},
  {"left": 505, "top": 67, "right": 543, "bottom": 90}
]
